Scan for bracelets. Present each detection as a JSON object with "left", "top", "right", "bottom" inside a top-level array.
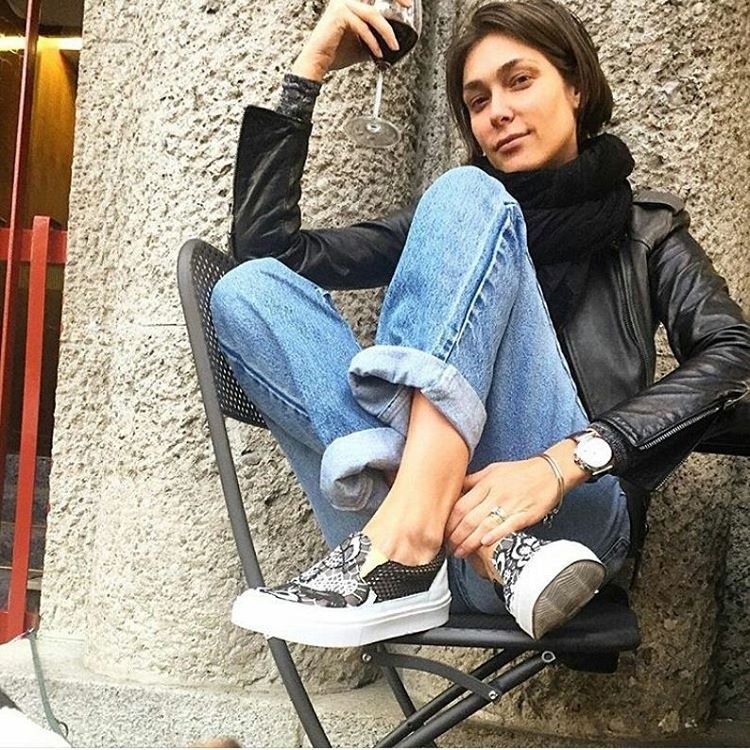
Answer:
[{"left": 534, "top": 454, "right": 564, "bottom": 524}]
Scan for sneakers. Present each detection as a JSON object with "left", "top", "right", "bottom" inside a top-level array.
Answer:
[
  {"left": 494, "top": 530, "right": 607, "bottom": 642},
  {"left": 231, "top": 531, "right": 452, "bottom": 648}
]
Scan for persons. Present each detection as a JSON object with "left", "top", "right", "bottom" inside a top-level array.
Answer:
[{"left": 210, "top": 0, "right": 750, "bottom": 648}]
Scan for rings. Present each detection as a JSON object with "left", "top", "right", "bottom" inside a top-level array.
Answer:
[{"left": 489, "top": 507, "right": 507, "bottom": 524}]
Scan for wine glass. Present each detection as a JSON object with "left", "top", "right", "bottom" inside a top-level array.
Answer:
[{"left": 343, "top": 0, "right": 422, "bottom": 148}]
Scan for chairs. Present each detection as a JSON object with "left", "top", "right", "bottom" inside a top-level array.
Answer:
[{"left": 177, "top": 238, "right": 640, "bottom": 747}]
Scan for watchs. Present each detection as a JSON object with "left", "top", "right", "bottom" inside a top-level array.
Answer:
[{"left": 566, "top": 430, "right": 614, "bottom": 484}]
